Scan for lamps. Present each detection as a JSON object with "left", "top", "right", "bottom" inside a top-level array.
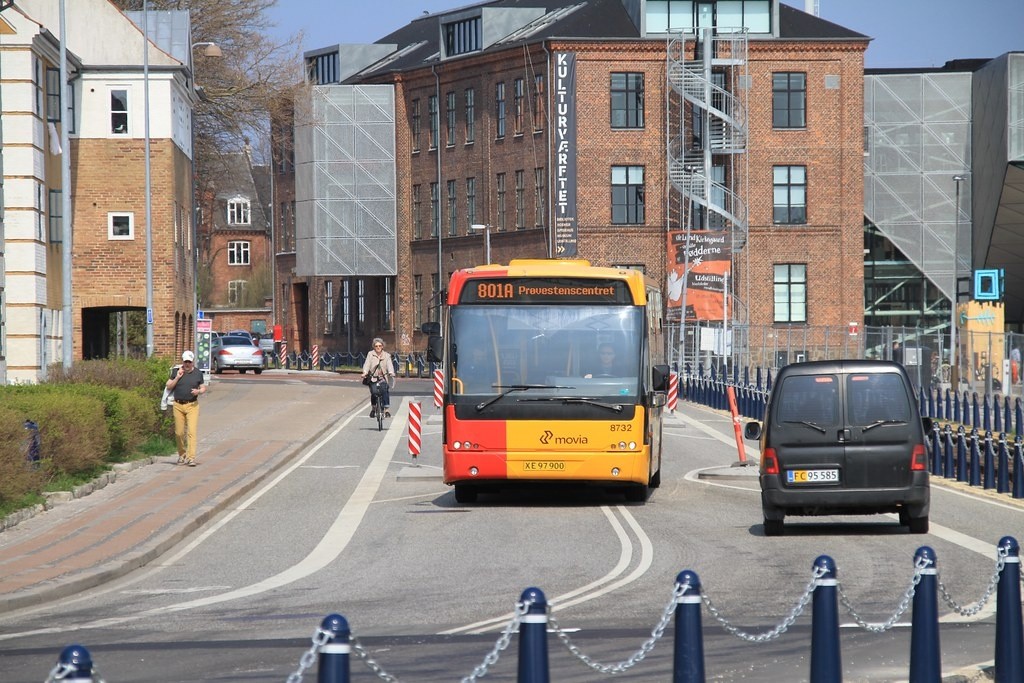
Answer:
[{"left": 193, "top": 41, "right": 222, "bottom": 57}]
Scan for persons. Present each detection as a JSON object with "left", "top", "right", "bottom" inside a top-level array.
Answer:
[
  {"left": 1011, "top": 344, "right": 1021, "bottom": 385},
  {"left": 361, "top": 337, "right": 395, "bottom": 418},
  {"left": 884, "top": 342, "right": 903, "bottom": 364},
  {"left": 166, "top": 351, "right": 205, "bottom": 465},
  {"left": 584, "top": 342, "right": 627, "bottom": 378}
]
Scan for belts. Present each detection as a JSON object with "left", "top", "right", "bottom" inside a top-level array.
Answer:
[{"left": 176, "top": 398, "right": 196, "bottom": 404}]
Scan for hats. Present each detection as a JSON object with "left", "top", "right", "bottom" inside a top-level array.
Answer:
[{"left": 181, "top": 351, "right": 194, "bottom": 362}]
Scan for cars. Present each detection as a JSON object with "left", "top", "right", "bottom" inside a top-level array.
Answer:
[
  {"left": 212, "top": 330, "right": 277, "bottom": 355},
  {"left": 211, "top": 335, "right": 264, "bottom": 375}
]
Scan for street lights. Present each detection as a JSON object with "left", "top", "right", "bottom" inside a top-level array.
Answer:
[
  {"left": 471, "top": 223, "right": 492, "bottom": 264},
  {"left": 950, "top": 173, "right": 966, "bottom": 392}
]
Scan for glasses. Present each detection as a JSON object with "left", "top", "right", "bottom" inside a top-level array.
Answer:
[
  {"left": 600, "top": 352, "right": 613, "bottom": 356},
  {"left": 374, "top": 345, "right": 383, "bottom": 347},
  {"left": 184, "top": 361, "right": 192, "bottom": 363}
]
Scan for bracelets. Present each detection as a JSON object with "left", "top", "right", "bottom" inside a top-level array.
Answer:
[{"left": 199, "top": 390, "right": 200, "bottom": 394}]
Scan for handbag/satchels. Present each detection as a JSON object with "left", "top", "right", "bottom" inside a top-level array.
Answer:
[{"left": 363, "top": 376, "right": 372, "bottom": 385}]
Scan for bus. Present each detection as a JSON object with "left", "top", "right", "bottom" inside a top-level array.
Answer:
[{"left": 421, "top": 260, "right": 671, "bottom": 503}]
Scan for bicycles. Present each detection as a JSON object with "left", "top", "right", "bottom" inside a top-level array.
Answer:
[{"left": 363, "top": 372, "right": 388, "bottom": 432}]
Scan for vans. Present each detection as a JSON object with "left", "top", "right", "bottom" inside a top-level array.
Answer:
[{"left": 743, "top": 357, "right": 934, "bottom": 535}]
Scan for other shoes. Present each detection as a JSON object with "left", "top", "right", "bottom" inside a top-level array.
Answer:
[
  {"left": 177, "top": 454, "right": 186, "bottom": 465},
  {"left": 385, "top": 412, "right": 390, "bottom": 418},
  {"left": 370, "top": 410, "right": 375, "bottom": 418},
  {"left": 188, "top": 459, "right": 196, "bottom": 466}
]
